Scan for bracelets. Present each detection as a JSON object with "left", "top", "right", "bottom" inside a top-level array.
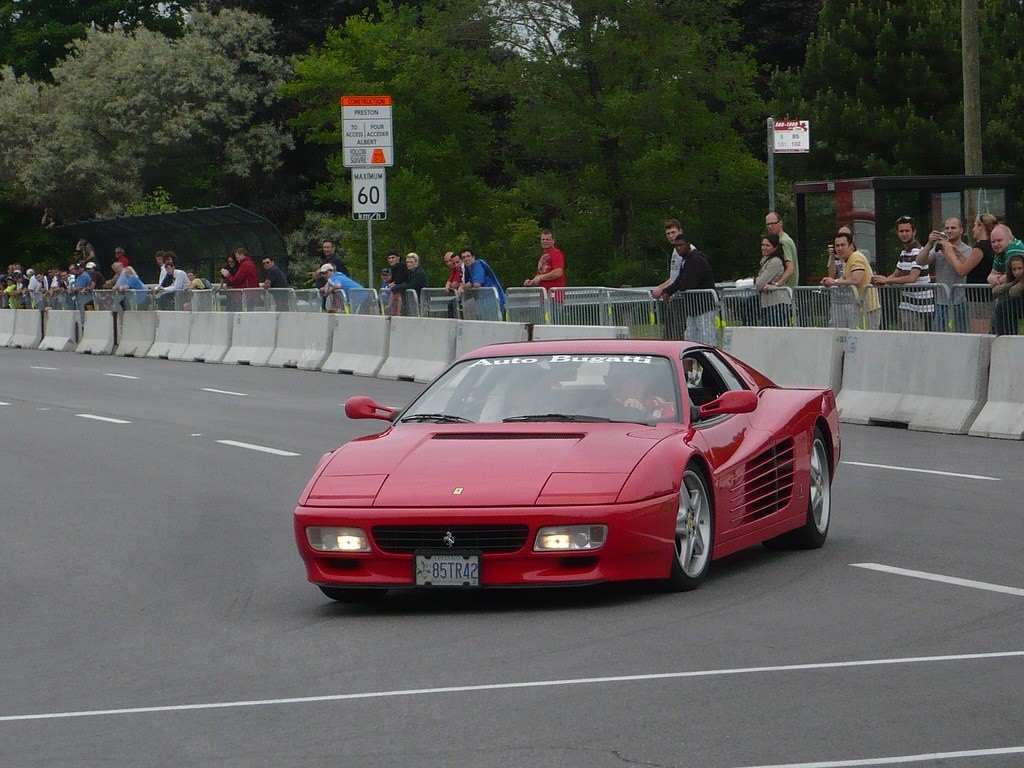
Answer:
[{"left": 776, "top": 283, "right": 780, "bottom": 287}]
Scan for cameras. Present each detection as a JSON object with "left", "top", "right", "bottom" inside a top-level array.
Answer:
[{"left": 936, "top": 231, "right": 946, "bottom": 239}]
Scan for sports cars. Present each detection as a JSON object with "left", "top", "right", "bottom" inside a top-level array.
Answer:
[{"left": 293, "top": 337, "right": 843, "bottom": 602}]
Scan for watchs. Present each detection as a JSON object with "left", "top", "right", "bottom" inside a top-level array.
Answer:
[{"left": 835, "top": 279, "right": 839, "bottom": 286}]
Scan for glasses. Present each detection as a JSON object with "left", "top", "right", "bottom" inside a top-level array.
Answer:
[
  {"left": 603, "top": 375, "right": 622, "bottom": 385},
  {"left": 896, "top": 215, "right": 914, "bottom": 225},
  {"left": 765, "top": 219, "right": 780, "bottom": 226},
  {"left": 319, "top": 269, "right": 332, "bottom": 276},
  {"left": 262, "top": 261, "right": 271, "bottom": 265}
]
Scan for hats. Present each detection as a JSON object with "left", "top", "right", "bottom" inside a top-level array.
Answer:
[
  {"left": 380, "top": 267, "right": 392, "bottom": 276},
  {"left": 85, "top": 262, "right": 96, "bottom": 268}
]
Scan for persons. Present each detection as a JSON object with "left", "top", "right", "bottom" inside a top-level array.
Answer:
[
  {"left": 217, "top": 249, "right": 261, "bottom": 311},
  {"left": 822, "top": 233, "right": 881, "bottom": 329},
  {"left": 524, "top": 229, "right": 566, "bottom": 324},
  {"left": 479, "top": 371, "right": 540, "bottom": 423},
  {"left": 445, "top": 248, "right": 506, "bottom": 321},
  {"left": 987, "top": 224, "right": 1024, "bottom": 335},
  {"left": 263, "top": 255, "right": 288, "bottom": 311},
  {"left": 0, "top": 235, "right": 149, "bottom": 310},
  {"left": 916, "top": 217, "right": 973, "bottom": 332},
  {"left": 380, "top": 250, "right": 427, "bottom": 317},
  {"left": 609, "top": 363, "right": 674, "bottom": 420},
  {"left": 755, "top": 212, "right": 798, "bottom": 327},
  {"left": 939, "top": 214, "right": 999, "bottom": 333},
  {"left": 872, "top": 218, "right": 935, "bottom": 331},
  {"left": 827, "top": 225, "right": 870, "bottom": 328},
  {"left": 315, "top": 239, "right": 373, "bottom": 315},
  {"left": 151, "top": 252, "right": 209, "bottom": 311},
  {"left": 652, "top": 219, "right": 714, "bottom": 341}
]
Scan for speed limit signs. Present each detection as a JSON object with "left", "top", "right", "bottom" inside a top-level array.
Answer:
[{"left": 350, "top": 169, "right": 387, "bottom": 222}]
